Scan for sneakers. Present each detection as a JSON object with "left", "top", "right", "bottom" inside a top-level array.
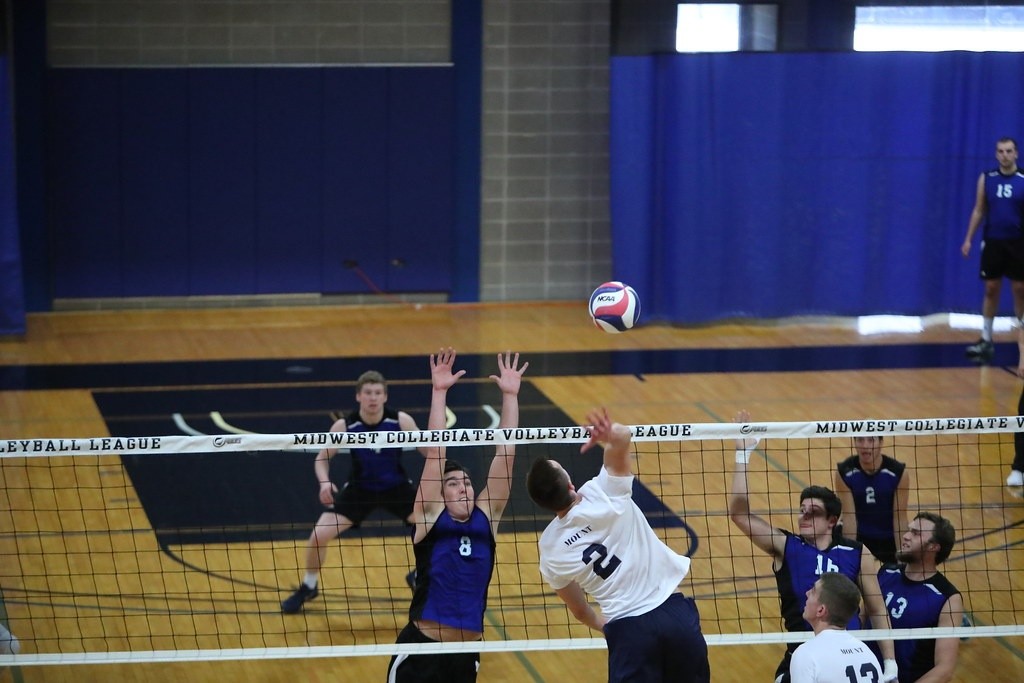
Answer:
[
  {"left": 406, "top": 568, "right": 417, "bottom": 596},
  {"left": 965, "top": 337, "right": 995, "bottom": 354},
  {"left": 281, "top": 582, "right": 320, "bottom": 613},
  {"left": 1007, "top": 471, "right": 1023, "bottom": 487}
]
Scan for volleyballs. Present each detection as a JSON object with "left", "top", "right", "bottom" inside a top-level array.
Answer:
[{"left": 588, "top": 278, "right": 644, "bottom": 334}]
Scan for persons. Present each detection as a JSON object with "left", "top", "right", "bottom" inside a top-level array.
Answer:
[
  {"left": 727, "top": 409, "right": 963, "bottom": 683},
  {"left": 387, "top": 349, "right": 530, "bottom": 683},
  {"left": 962, "top": 137, "right": 1024, "bottom": 357},
  {"left": 527, "top": 405, "right": 710, "bottom": 683},
  {"left": 282, "top": 370, "right": 428, "bottom": 614},
  {"left": 1009, "top": 317, "right": 1024, "bottom": 489}
]
